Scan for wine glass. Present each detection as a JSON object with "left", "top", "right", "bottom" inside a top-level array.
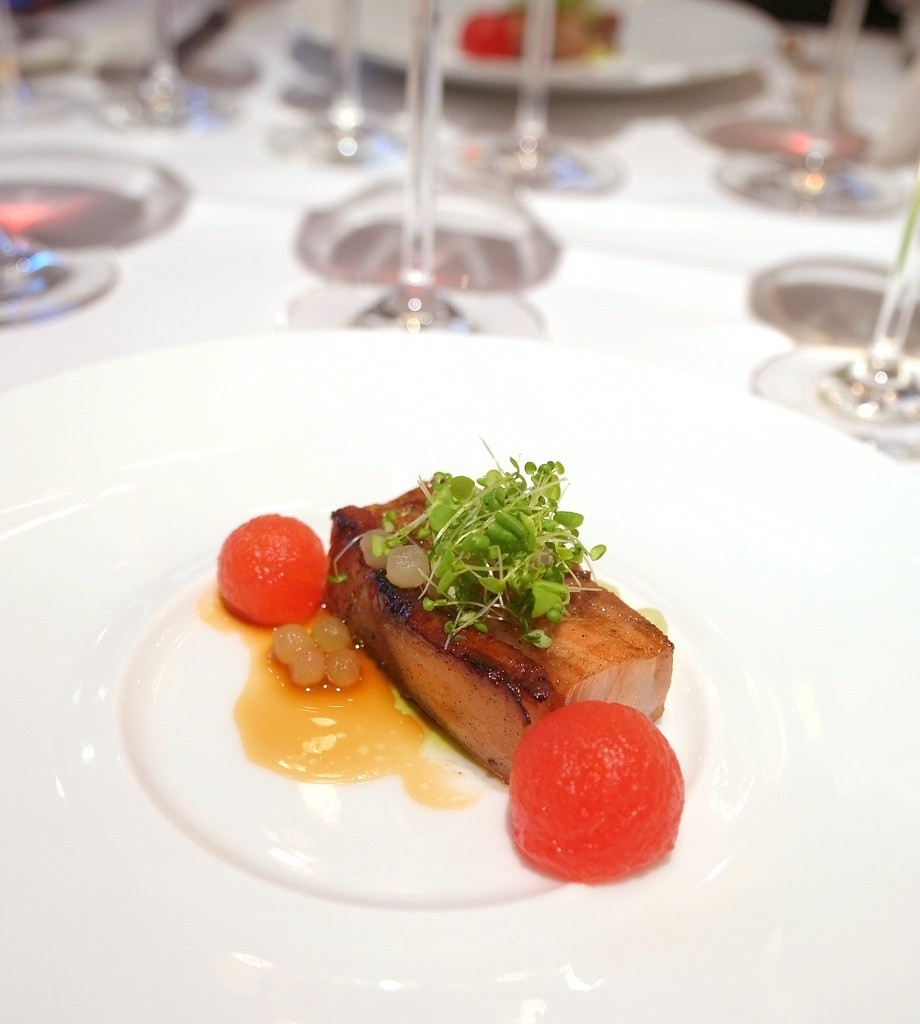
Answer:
[
  {"left": 101, "top": 0, "right": 226, "bottom": 132},
  {"left": 0, "top": 227, "right": 120, "bottom": 326},
  {"left": 450, "top": 0, "right": 629, "bottom": 197},
  {"left": 346, "top": 2, "right": 488, "bottom": 330},
  {"left": 301, "top": 0, "right": 398, "bottom": 184},
  {"left": 749, "top": 192, "right": 919, "bottom": 475},
  {"left": 294, "top": 0, "right": 563, "bottom": 290},
  {"left": 679, "top": 0, "right": 919, "bottom": 218}
]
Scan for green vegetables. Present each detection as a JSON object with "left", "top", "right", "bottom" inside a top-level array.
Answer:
[{"left": 330, "top": 437, "right": 606, "bottom": 650}]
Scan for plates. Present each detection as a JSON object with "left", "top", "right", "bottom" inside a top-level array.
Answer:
[
  {"left": 289, "top": 0, "right": 778, "bottom": 91},
  {"left": 3, "top": 330, "right": 919, "bottom": 1024}
]
